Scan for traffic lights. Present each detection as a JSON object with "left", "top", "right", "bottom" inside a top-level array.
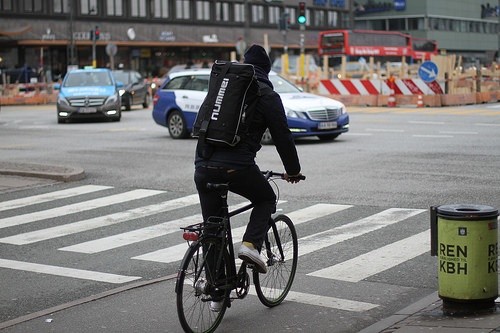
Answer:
[
  {"left": 284, "top": 14, "right": 292, "bottom": 32},
  {"left": 297, "top": 2, "right": 306, "bottom": 25},
  {"left": 95, "top": 25, "right": 100, "bottom": 40}
]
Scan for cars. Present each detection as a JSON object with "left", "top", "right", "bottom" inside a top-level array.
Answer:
[
  {"left": 152, "top": 67, "right": 350, "bottom": 146},
  {"left": 54, "top": 68, "right": 124, "bottom": 124},
  {"left": 144, "top": 62, "right": 212, "bottom": 98},
  {"left": 111, "top": 69, "right": 150, "bottom": 111}
]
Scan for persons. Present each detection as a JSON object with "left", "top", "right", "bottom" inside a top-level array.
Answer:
[{"left": 192, "top": 44, "right": 303, "bottom": 313}]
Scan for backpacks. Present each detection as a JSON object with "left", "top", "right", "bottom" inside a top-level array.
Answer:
[{"left": 186, "top": 58, "right": 272, "bottom": 159}]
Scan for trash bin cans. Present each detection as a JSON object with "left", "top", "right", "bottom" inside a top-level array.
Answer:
[{"left": 428, "top": 205, "right": 500, "bottom": 316}]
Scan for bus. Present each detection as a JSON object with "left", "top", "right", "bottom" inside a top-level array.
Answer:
[
  {"left": 318, "top": 29, "right": 412, "bottom": 79},
  {"left": 411, "top": 36, "right": 438, "bottom": 63}
]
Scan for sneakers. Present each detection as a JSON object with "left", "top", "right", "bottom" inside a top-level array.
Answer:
[
  {"left": 237, "top": 244, "right": 269, "bottom": 275},
  {"left": 207, "top": 299, "right": 224, "bottom": 312}
]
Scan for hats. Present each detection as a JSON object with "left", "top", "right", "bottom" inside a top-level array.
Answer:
[{"left": 243, "top": 45, "right": 271, "bottom": 74}]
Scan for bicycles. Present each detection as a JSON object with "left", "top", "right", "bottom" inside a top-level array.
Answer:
[{"left": 175, "top": 170, "right": 306, "bottom": 333}]
